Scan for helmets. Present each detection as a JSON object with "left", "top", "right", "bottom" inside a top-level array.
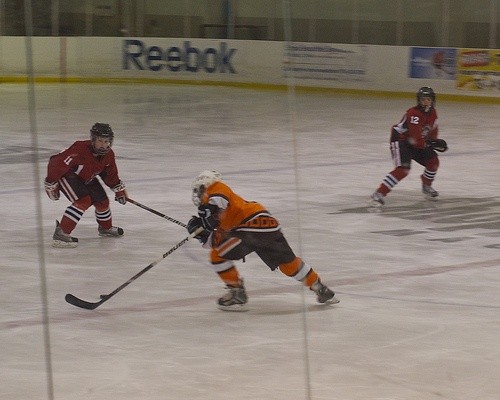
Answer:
[
  {"left": 90, "top": 122, "right": 113, "bottom": 138},
  {"left": 416, "top": 86, "right": 436, "bottom": 98},
  {"left": 192, "top": 169, "right": 222, "bottom": 189}
]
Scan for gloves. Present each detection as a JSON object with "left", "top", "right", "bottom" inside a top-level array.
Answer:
[
  {"left": 45, "top": 188, "right": 60, "bottom": 200},
  {"left": 187, "top": 216, "right": 219, "bottom": 244},
  {"left": 115, "top": 189, "right": 127, "bottom": 205}
]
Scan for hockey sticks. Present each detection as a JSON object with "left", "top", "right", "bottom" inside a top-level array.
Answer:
[
  {"left": 64, "top": 225, "right": 204, "bottom": 311},
  {"left": 126, "top": 197, "right": 188, "bottom": 230}
]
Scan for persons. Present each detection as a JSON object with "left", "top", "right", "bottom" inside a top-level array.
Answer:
[
  {"left": 367, "top": 87, "right": 448, "bottom": 208},
  {"left": 45, "top": 123, "right": 127, "bottom": 248},
  {"left": 187, "top": 171, "right": 340, "bottom": 312}
]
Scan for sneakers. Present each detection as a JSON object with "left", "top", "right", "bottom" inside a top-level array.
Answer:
[
  {"left": 51, "top": 219, "right": 79, "bottom": 248},
  {"left": 367, "top": 192, "right": 385, "bottom": 208},
  {"left": 422, "top": 183, "right": 438, "bottom": 201},
  {"left": 96, "top": 219, "right": 124, "bottom": 237},
  {"left": 217, "top": 284, "right": 248, "bottom": 312},
  {"left": 317, "top": 284, "right": 341, "bottom": 305}
]
